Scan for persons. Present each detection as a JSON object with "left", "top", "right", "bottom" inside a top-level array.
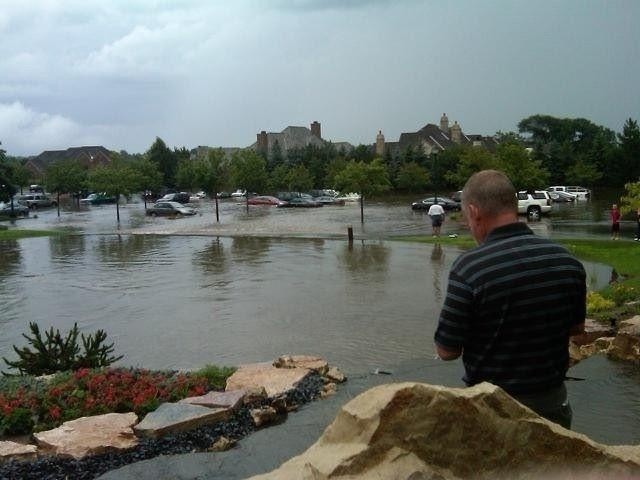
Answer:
[
  {"left": 610, "top": 204, "right": 621, "bottom": 240},
  {"left": 436, "top": 170, "right": 586, "bottom": 432},
  {"left": 633, "top": 206, "right": 640, "bottom": 241},
  {"left": 428, "top": 198, "right": 446, "bottom": 239}
]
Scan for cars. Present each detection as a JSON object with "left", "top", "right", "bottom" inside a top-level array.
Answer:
[{"left": 411, "top": 183, "right": 592, "bottom": 222}]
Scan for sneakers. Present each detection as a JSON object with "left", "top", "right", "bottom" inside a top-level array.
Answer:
[
  {"left": 432, "top": 235, "right": 440, "bottom": 239},
  {"left": 610, "top": 237, "right": 620, "bottom": 241}
]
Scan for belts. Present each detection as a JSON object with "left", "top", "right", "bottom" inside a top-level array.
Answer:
[
  {"left": 465, "top": 376, "right": 566, "bottom": 395},
  {"left": 432, "top": 215, "right": 441, "bottom": 216}
]
